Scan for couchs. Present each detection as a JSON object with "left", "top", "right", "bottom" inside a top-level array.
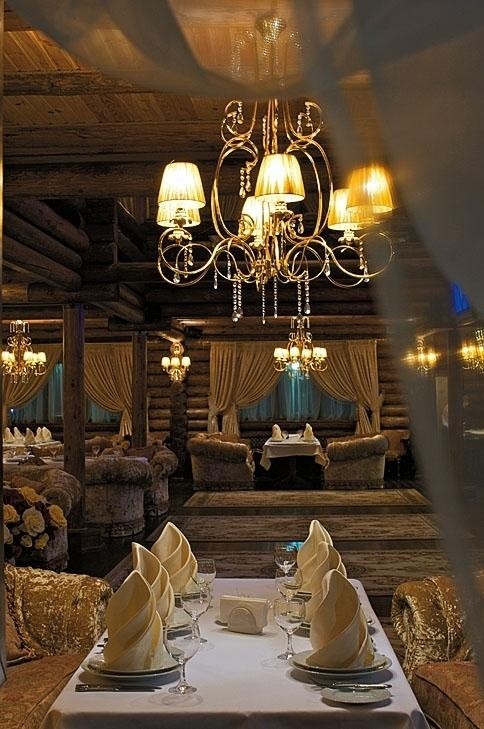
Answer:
[
  {"left": 392, "top": 560, "right": 484, "bottom": 729},
  {"left": 322, "top": 434, "right": 389, "bottom": 490},
  {"left": 188, "top": 430, "right": 255, "bottom": 491},
  {"left": 58, "top": 454, "right": 149, "bottom": 539},
  {"left": 79, "top": 432, "right": 131, "bottom": 467},
  {"left": 100, "top": 445, "right": 179, "bottom": 514},
  {"left": 0, "top": 561, "right": 131, "bottom": 729},
  {"left": 0, "top": 463, "right": 79, "bottom": 576}
]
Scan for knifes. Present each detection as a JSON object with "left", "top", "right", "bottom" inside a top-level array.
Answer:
[{"left": 74, "top": 682, "right": 161, "bottom": 694}]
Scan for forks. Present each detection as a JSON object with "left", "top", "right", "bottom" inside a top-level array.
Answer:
[{"left": 306, "top": 673, "right": 392, "bottom": 690}]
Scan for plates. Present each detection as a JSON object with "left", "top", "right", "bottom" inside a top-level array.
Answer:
[
  {"left": 287, "top": 649, "right": 393, "bottom": 679},
  {"left": 294, "top": 651, "right": 388, "bottom": 672},
  {"left": 320, "top": 685, "right": 391, "bottom": 704},
  {"left": 80, "top": 660, "right": 186, "bottom": 682},
  {"left": 89, "top": 654, "right": 181, "bottom": 676}
]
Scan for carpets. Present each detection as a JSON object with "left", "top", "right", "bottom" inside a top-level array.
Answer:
[
  {"left": 182, "top": 488, "right": 431, "bottom": 506},
  {"left": 146, "top": 513, "right": 473, "bottom": 543},
  {"left": 103, "top": 547, "right": 484, "bottom": 596}
]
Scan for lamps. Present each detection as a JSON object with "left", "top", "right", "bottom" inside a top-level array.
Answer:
[
  {"left": 0, "top": 319, "right": 46, "bottom": 380},
  {"left": 457, "top": 320, "right": 484, "bottom": 373},
  {"left": 405, "top": 320, "right": 455, "bottom": 375},
  {"left": 267, "top": 317, "right": 327, "bottom": 378},
  {"left": 162, "top": 342, "right": 191, "bottom": 381},
  {"left": 152, "top": 12, "right": 396, "bottom": 327}
]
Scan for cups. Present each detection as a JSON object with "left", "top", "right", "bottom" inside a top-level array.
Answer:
[
  {"left": 273, "top": 544, "right": 299, "bottom": 575},
  {"left": 272, "top": 597, "right": 307, "bottom": 662},
  {"left": 162, "top": 623, "right": 202, "bottom": 697},
  {"left": 190, "top": 559, "right": 217, "bottom": 613},
  {"left": 273, "top": 568, "right": 303, "bottom": 618},
  {"left": 179, "top": 584, "right": 211, "bottom": 643}
]
